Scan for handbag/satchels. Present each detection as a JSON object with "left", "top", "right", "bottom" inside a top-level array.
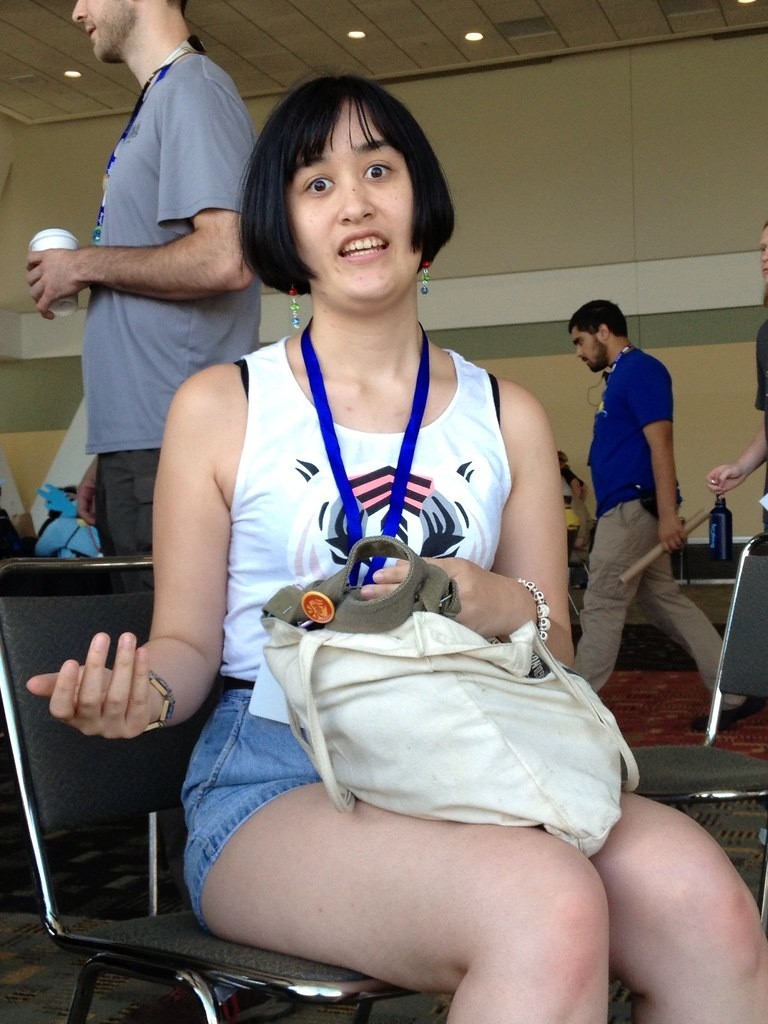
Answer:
[{"left": 261, "top": 537, "right": 640, "bottom": 859}]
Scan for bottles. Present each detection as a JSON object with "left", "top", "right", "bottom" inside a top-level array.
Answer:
[{"left": 708, "top": 493, "right": 733, "bottom": 561}]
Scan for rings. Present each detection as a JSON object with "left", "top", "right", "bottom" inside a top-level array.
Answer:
[{"left": 711, "top": 480, "right": 714, "bottom": 484}]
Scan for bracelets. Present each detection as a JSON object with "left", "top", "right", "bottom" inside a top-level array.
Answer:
[
  {"left": 487, "top": 578, "right": 551, "bottom": 647},
  {"left": 142, "top": 669, "right": 177, "bottom": 732}
]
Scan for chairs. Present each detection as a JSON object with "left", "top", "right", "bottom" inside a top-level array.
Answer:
[
  {"left": 0, "top": 555, "right": 444, "bottom": 1024},
  {"left": 616, "top": 530, "right": 767, "bottom": 928}
]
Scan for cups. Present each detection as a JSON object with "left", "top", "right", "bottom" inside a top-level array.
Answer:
[{"left": 28, "top": 228, "right": 80, "bottom": 317}]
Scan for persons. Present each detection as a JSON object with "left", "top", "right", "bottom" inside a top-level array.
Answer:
[
  {"left": 555, "top": 450, "right": 589, "bottom": 589},
  {"left": 25, "top": 0, "right": 262, "bottom": 558},
  {"left": 569, "top": 299, "right": 765, "bottom": 732},
  {"left": 0, "top": 481, "right": 103, "bottom": 558},
  {"left": 705, "top": 219, "right": 768, "bottom": 533},
  {"left": 26, "top": 72, "right": 768, "bottom": 1024}
]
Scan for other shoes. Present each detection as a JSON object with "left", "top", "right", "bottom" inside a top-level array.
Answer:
[{"left": 691, "top": 695, "right": 766, "bottom": 734}]
